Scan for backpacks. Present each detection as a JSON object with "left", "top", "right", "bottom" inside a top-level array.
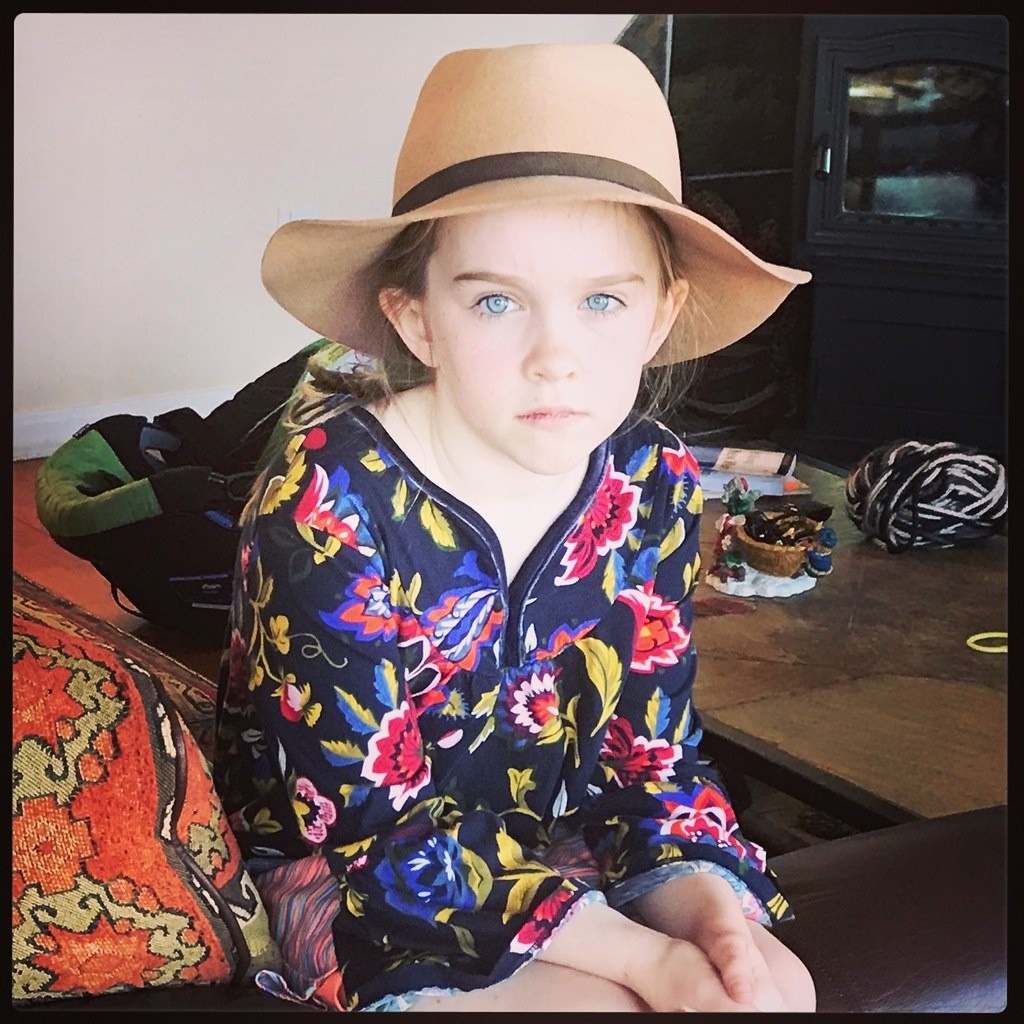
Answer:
[{"left": 32, "top": 331, "right": 333, "bottom": 648}]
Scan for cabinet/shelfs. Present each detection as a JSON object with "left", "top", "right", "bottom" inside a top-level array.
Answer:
[{"left": 790, "top": 17, "right": 1011, "bottom": 474}]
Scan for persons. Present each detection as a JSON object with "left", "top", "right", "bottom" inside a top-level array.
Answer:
[{"left": 208, "top": 43, "right": 823, "bottom": 1012}]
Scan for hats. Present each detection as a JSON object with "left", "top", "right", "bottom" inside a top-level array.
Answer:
[{"left": 260, "top": 41, "right": 812, "bottom": 369}]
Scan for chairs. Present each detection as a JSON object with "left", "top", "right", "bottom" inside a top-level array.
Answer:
[{"left": 12, "top": 571, "right": 290, "bottom": 1009}]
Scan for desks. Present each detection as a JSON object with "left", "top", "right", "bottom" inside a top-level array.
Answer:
[{"left": 694, "top": 464, "right": 1007, "bottom": 850}]
